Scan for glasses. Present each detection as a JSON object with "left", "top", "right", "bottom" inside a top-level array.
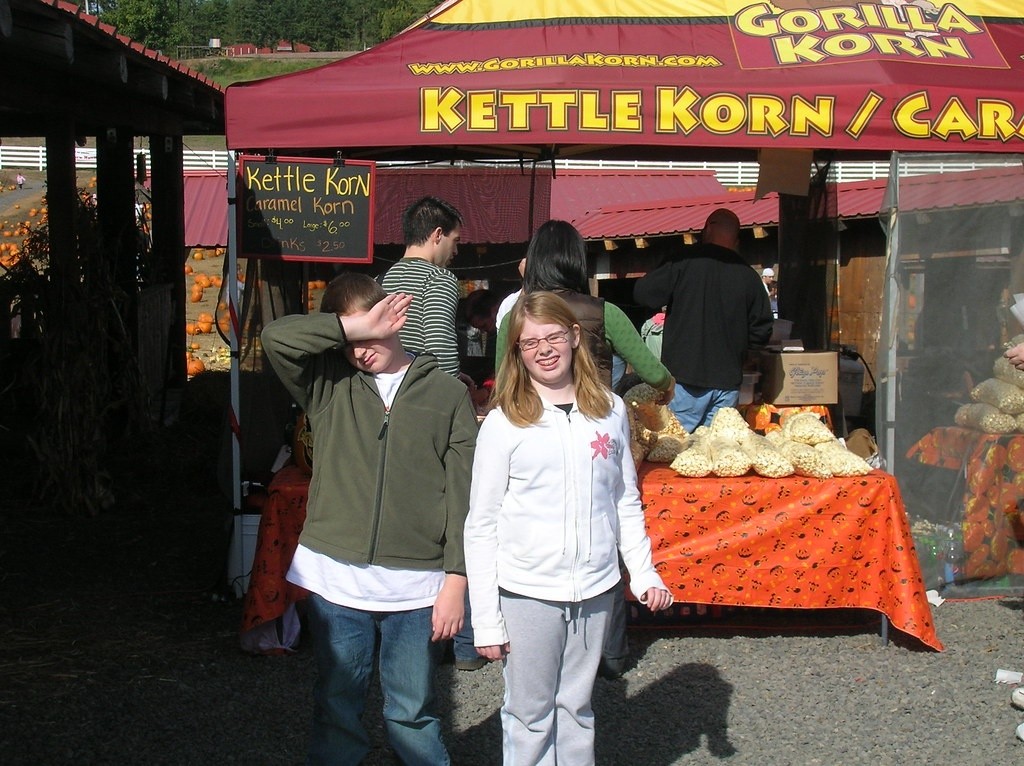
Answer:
[{"left": 514, "top": 327, "right": 570, "bottom": 350}]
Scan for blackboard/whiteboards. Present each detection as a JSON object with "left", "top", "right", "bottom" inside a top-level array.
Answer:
[{"left": 234, "top": 153, "right": 377, "bottom": 265}]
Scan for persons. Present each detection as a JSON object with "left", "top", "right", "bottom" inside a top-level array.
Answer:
[
  {"left": 462, "top": 292, "right": 675, "bottom": 766},
  {"left": 634, "top": 209, "right": 785, "bottom": 435},
  {"left": 374, "top": 195, "right": 510, "bottom": 406},
  {"left": 761, "top": 268, "right": 778, "bottom": 319},
  {"left": 641, "top": 305, "right": 667, "bottom": 363},
  {"left": 261, "top": 272, "right": 478, "bottom": 766},
  {"left": 905, "top": 258, "right": 1024, "bottom": 513},
  {"left": 495, "top": 219, "right": 675, "bottom": 407}
]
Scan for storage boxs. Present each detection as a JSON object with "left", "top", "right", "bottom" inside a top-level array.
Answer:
[{"left": 737, "top": 317, "right": 863, "bottom": 418}]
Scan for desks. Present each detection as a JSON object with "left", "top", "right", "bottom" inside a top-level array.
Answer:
[
  {"left": 907, "top": 426, "right": 1024, "bottom": 584},
  {"left": 737, "top": 404, "right": 835, "bottom": 437},
  {"left": 238, "top": 462, "right": 945, "bottom": 668}
]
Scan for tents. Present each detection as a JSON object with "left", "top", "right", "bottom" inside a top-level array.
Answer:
[{"left": 223, "top": 0, "right": 1024, "bottom": 607}]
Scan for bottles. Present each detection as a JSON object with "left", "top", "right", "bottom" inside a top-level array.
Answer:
[{"left": 918, "top": 525, "right": 959, "bottom": 585}]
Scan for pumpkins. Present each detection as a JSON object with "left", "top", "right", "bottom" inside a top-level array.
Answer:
[
  {"left": 0, "top": 176, "right": 154, "bottom": 269},
  {"left": 183, "top": 248, "right": 324, "bottom": 376}
]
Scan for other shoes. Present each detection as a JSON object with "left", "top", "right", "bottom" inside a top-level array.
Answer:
[
  {"left": 457, "top": 656, "right": 489, "bottom": 669},
  {"left": 602, "top": 656, "right": 625, "bottom": 680}
]
